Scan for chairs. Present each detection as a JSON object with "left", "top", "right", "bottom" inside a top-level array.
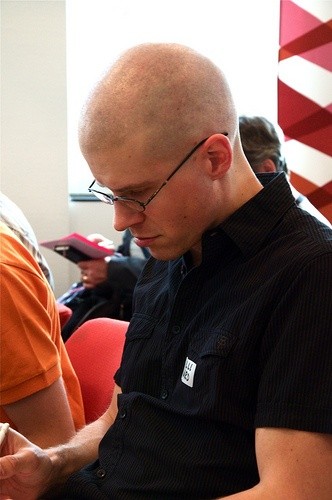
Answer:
[{"left": 64, "top": 318, "right": 130, "bottom": 424}]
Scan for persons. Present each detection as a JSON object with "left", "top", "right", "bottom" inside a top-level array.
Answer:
[
  {"left": 0, "top": 191, "right": 53, "bottom": 292},
  {"left": 0, "top": 232, "right": 89, "bottom": 500},
  {"left": 56, "top": 229, "right": 153, "bottom": 344},
  {"left": 0, "top": 44, "right": 332, "bottom": 499},
  {"left": 238, "top": 117, "right": 332, "bottom": 229}
]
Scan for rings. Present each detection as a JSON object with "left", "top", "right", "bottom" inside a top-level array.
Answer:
[{"left": 83, "top": 276, "right": 88, "bottom": 283}]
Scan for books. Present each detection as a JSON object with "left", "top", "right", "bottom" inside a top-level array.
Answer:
[{"left": 54, "top": 245, "right": 92, "bottom": 264}]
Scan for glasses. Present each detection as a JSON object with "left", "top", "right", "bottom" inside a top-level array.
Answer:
[{"left": 88, "top": 132, "right": 230, "bottom": 212}]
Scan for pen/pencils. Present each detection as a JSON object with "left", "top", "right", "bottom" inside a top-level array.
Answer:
[{"left": 0, "top": 423, "right": 10, "bottom": 444}]
